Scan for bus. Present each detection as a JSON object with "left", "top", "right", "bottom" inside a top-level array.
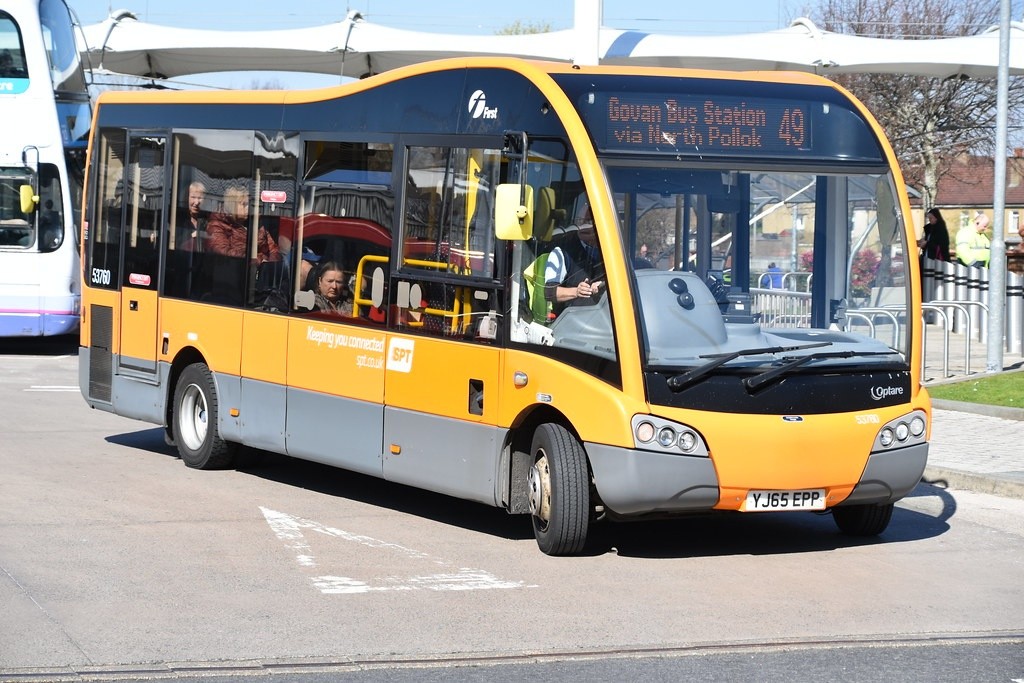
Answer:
[
  {"left": 77, "top": 57, "right": 935, "bottom": 557},
  {"left": 1, "top": 0, "right": 96, "bottom": 341}
]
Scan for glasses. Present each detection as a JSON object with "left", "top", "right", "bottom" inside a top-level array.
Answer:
[{"left": 574, "top": 218, "right": 594, "bottom": 227}]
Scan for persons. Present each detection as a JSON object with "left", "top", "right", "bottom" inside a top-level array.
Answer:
[
  {"left": 916, "top": 209, "right": 951, "bottom": 263},
  {"left": 107, "top": 178, "right": 139, "bottom": 247},
  {"left": 760, "top": 262, "right": 788, "bottom": 290},
  {"left": 638, "top": 244, "right": 658, "bottom": 270},
  {"left": 955, "top": 215, "right": 992, "bottom": 268},
  {"left": 172, "top": 181, "right": 211, "bottom": 252},
  {"left": 40, "top": 199, "right": 62, "bottom": 248},
  {"left": 344, "top": 270, "right": 369, "bottom": 316},
  {"left": 305, "top": 260, "right": 366, "bottom": 320},
  {"left": 206, "top": 182, "right": 281, "bottom": 276},
  {"left": 0, "top": 55, "right": 23, "bottom": 78},
  {"left": 688, "top": 249, "right": 699, "bottom": 273},
  {"left": 547, "top": 201, "right": 608, "bottom": 317},
  {"left": 280, "top": 201, "right": 296, "bottom": 261}
]
